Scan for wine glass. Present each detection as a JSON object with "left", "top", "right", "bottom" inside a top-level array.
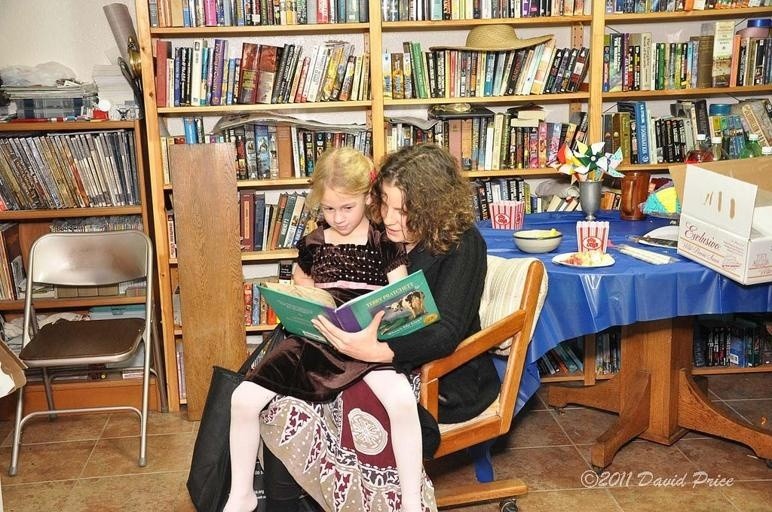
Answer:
[{"left": 578, "top": 181, "right": 604, "bottom": 220}]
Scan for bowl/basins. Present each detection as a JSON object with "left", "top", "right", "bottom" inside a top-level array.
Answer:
[{"left": 512, "top": 229, "right": 562, "bottom": 253}]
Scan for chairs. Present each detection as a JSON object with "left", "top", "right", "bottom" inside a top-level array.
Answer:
[
  {"left": 409, "top": 255, "right": 550, "bottom": 510},
  {"left": 9, "top": 230, "right": 154, "bottom": 476}
]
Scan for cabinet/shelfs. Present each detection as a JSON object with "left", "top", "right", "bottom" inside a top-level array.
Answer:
[
  {"left": 0, "top": 119, "right": 163, "bottom": 421},
  {"left": 134, "top": 0, "right": 771, "bottom": 415}
]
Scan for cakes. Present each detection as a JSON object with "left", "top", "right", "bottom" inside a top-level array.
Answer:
[
  {"left": 640, "top": 179, "right": 681, "bottom": 215},
  {"left": 569, "top": 250, "right": 602, "bottom": 266}
]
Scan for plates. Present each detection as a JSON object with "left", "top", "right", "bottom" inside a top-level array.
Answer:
[{"left": 552, "top": 252, "right": 615, "bottom": 269}]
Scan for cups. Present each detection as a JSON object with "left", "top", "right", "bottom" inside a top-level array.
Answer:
[{"left": 621, "top": 170, "right": 650, "bottom": 221}]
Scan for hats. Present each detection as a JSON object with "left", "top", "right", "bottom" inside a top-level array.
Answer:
[{"left": 430, "top": 24, "right": 554, "bottom": 51}]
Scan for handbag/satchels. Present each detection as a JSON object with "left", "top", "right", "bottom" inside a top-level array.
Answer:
[{"left": 187, "top": 365, "right": 244, "bottom": 512}]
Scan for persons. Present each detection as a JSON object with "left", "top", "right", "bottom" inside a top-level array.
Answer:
[
  {"left": 223, "top": 147, "right": 425, "bottom": 512},
  {"left": 262, "top": 143, "right": 501, "bottom": 512}
]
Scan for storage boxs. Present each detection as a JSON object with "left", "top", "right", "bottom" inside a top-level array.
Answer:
[{"left": 666, "top": 154, "right": 771, "bottom": 286}]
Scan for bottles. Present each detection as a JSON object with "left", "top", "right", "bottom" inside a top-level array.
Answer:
[{"left": 684, "top": 132, "right": 772, "bottom": 165}]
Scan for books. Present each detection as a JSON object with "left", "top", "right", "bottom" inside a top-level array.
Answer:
[{"left": 0, "top": 0, "right": 772, "bottom": 404}]
